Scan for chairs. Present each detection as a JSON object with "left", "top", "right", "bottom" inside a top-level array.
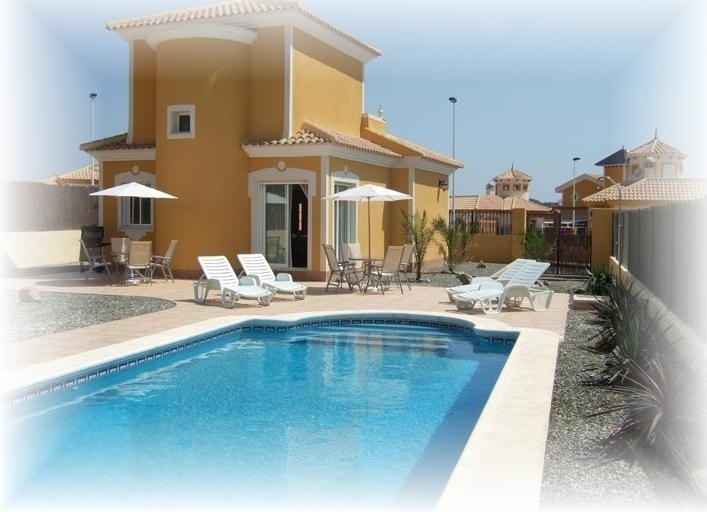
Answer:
[
  {"left": 78, "top": 223, "right": 179, "bottom": 285},
  {"left": 191, "top": 252, "right": 276, "bottom": 310},
  {"left": 447, "top": 258, "right": 557, "bottom": 315},
  {"left": 321, "top": 240, "right": 414, "bottom": 295},
  {"left": 235, "top": 254, "right": 306, "bottom": 302}
]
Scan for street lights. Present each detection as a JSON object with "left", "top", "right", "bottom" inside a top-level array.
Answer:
[
  {"left": 570, "top": 155, "right": 580, "bottom": 230},
  {"left": 595, "top": 175, "right": 621, "bottom": 288},
  {"left": 447, "top": 96, "right": 460, "bottom": 230},
  {"left": 89, "top": 90, "right": 99, "bottom": 191}
]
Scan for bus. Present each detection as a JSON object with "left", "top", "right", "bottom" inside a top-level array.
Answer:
[{"left": 544, "top": 220, "right": 576, "bottom": 228}]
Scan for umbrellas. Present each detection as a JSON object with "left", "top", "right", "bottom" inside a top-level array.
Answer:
[
  {"left": 88, "top": 181, "right": 178, "bottom": 224},
  {"left": 320, "top": 183, "right": 413, "bottom": 274},
  {"left": 266, "top": 191, "right": 286, "bottom": 204}
]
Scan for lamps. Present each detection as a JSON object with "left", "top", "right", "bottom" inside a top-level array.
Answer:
[{"left": 438, "top": 177, "right": 448, "bottom": 193}]
